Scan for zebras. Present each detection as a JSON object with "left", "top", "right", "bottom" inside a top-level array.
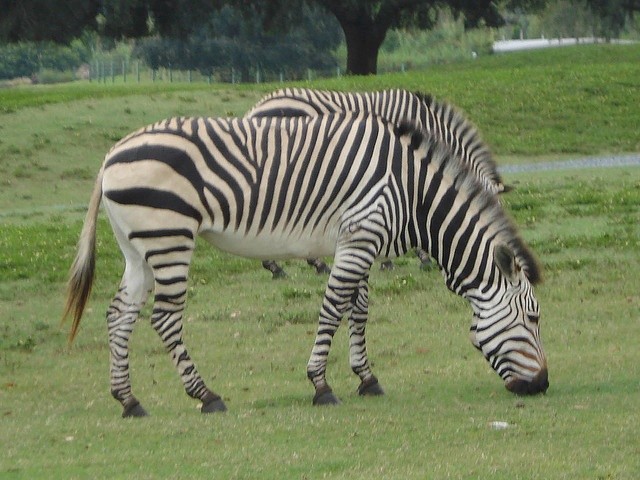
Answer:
[
  {"left": 58, "top": 110, "right": 550, "bottom": 419},
  {"left": 238, "top": 85, "right": 515, "bottom": 282}
]
